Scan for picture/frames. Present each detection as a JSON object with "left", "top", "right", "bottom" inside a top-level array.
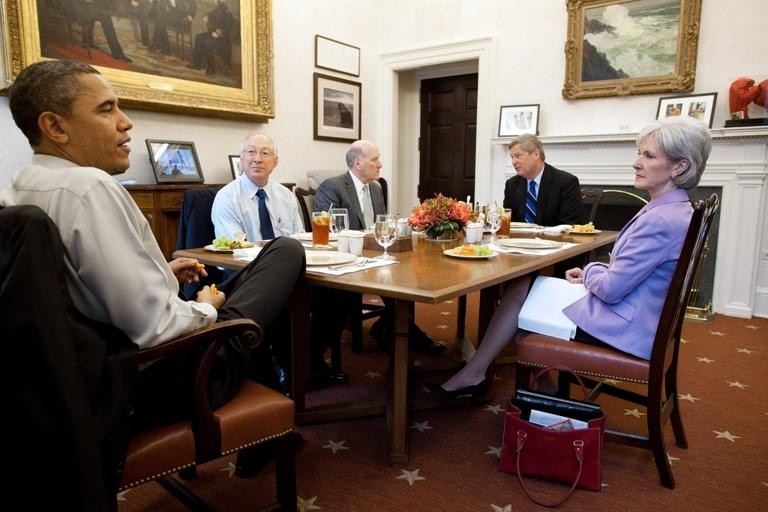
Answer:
[
  {"left": 497, "top": 103, "right": 540, "bottom": 135},
  {"left": 313, "top": 72, "right": 362, "bottom": 142},
  {"left": 314, "top": 34, "right": 360, "bottom": 77},
  {"left": 655, "top": 92, "right": 718, "bottom": 129},
  {"left": 228, "top": 155, "right": 243, "bottom": 180},
  {"left": 146, "top": 136, "right": 205, "bottom": 185},
  {"left": 0, "top": 0, "right": 276, "bottom": 121},
  {"left": 562, "top": 0, "right": 702, "bottom": 101}
]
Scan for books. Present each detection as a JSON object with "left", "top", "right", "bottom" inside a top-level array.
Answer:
[{"left": 510, "top": 386, "right": 602, "bottom": 430}]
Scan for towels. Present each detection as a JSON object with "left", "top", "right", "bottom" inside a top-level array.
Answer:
[
  {"left": 485, "top": 235, "right": 580, "bottom": 256},
  {"left": 231, "top": 245, "right": 400, "bottom": 276},
  {"left": 287, "top": 231, "right": 337, "bottom": 242},
  {"left": 510, "top": 223, "right": 574, "bottom": 232},
  {"left": 517, "top": 276, "right": 590, "bottom": 341}
]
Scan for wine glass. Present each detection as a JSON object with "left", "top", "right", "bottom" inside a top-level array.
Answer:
[
  {"left": 485, "top": 206, "right": 504, "bottom": 245},
  {"left": 374, "top": 215, "right": 399, "bottom": 260},
  {"left": 329, "top": 207, "right": 349, "bottom": 246},
  {"left": 464, "top": 200, "right": 512, "bottom": 238}
]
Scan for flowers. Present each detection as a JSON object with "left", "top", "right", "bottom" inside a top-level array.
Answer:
[{"left": 406, "top": 193, "right": 473, "bottom": 238}]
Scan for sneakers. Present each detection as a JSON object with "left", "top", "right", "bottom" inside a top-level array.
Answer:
[{"left": 235, "top": 430, "right": 305, "bottom": 480}]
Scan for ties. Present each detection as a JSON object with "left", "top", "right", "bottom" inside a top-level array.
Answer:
[
  {"left": 253, "top": 188, "right": 278, "bottom": 242},
  {"left": 522, "top": 180, "right": 538, "bottom": 225},
  {"left": 360, "top": 185, "right": 375, "bottom": 232}
]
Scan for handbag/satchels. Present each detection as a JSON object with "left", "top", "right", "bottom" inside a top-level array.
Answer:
[{"left": 494, "top": 364, "right": 608, "bottom": 510}]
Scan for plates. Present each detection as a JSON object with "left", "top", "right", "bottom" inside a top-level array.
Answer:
[
  {"left": 118, "top": 178, "right": 137, "bottom": 185},
  {"left": 564, "top": 228, "right": 602, "bottom": 234},
  {"left": 509, "top": 221, "right": 538, "bottom": 228},
  {"left": 443, "top": 248, "right": 499, "bottom": 258},
  {"left": 204, "top": 228, "right": 426, "bottom": 265},
  {"left": 498, "top": 237, "right": 564, "bottom": 249}
]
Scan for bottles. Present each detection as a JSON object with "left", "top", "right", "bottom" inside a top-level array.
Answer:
[
  {"left": 465, "top": 222, "right": 483, "bottom": 243},
  {"left": 397, "top": 218, "right": 414, "bottom": 236}
]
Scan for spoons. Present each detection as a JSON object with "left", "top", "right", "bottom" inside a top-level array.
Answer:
[{"left": 328, "top": 258, "right": 368, "bottom": 271}]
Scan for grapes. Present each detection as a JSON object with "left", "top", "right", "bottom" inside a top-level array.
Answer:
[{"left": 474, "top": 246, "right": 493, "bottom": 256}]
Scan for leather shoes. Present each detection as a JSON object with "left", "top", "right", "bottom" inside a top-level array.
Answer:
[
  {"left": 413, "top": 335, "right": 448, "bottom": 355},
  {"left": 421, "top": 379, "right": 487, "bottom": 403},
  {"left": 307, "top": 367, "right": 351, "bottom": 389}
]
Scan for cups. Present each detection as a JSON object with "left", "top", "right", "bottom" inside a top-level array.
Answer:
[{"left": 310, "top": 211, "right": 330, "bottom": 251}]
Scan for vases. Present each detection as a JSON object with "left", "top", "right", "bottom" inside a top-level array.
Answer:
[{"left": 428, "top": 231, "right": 458, "bottom": 240}]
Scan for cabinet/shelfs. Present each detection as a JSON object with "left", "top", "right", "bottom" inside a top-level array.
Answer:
[{"left": 121, "top": 182, "right": 296, "bottom": 262}]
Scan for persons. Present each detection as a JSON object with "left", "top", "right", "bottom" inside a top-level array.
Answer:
[
  {"left": 423, "top": 118, "right": 714, "bottom": 409},
  {"left": 177, "top": 150, "right": 188, "bottom": 168},
  {"left": 65, "top": 0, "right": 236, "bottom": 78},
  {"left": 209, "top": 131, "right": 350, "bottom": 384},
  {"left": 502, "top": 129, "right": 585, "bottom": 281},
  {"left": 313, "top": 140, "right": 450, "bottom": 356},
  {"left": 0, "top": 56, "right": 307, "bottom": 482},
  {"left": 171, "top": 165, "right": 184, "bottom": 174}
]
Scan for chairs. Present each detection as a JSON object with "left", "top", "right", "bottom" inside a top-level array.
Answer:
[
  {"left": 511, "top": 191, "right": 719, "bottom": 489},
  {"left": 0, "top": 205, "right": 298, "bottom": 512},
  {"left": 580, "top": 187, "right": 604, "bottom": 261},
  {"left": 175, "top": 187, "right": 224, "bottom": 298},
  {"left": 295, "top": 186, "right": 317, "bottom": 232}
]
